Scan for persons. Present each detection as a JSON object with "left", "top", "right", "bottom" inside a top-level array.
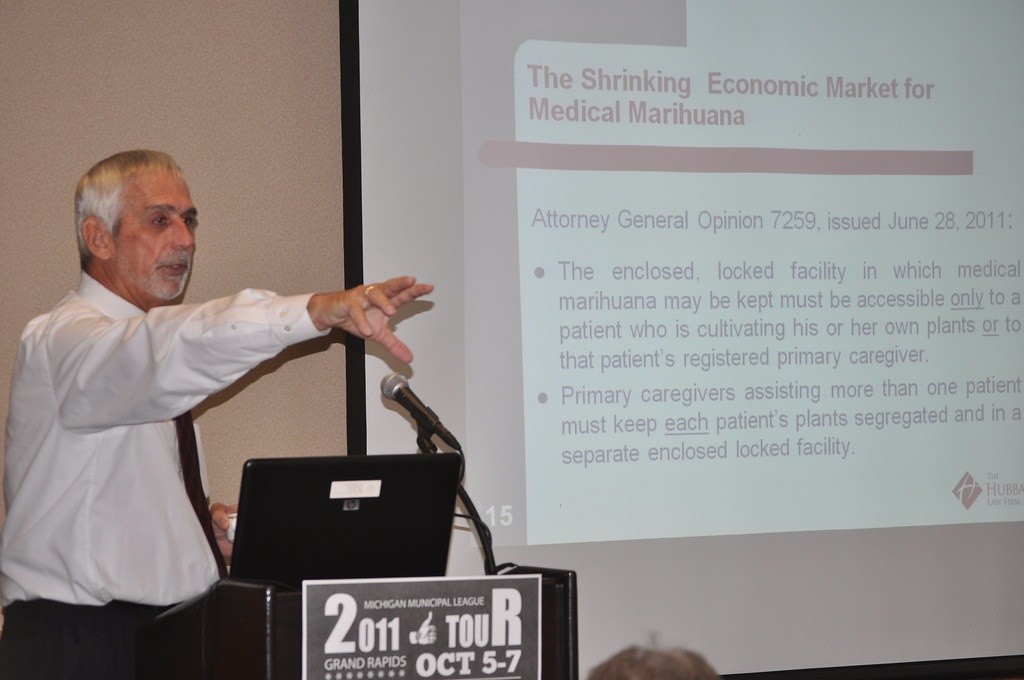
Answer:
[{"left": 0, "top": 147, "right": 432, "bottom": 679}]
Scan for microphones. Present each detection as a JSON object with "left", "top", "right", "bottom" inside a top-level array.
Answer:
[{"left": 379, "top": 372, "right": 463, "bottom": 448}]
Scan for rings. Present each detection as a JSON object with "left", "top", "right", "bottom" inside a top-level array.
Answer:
[{"left": 364, "top": 285, "right": 375, "bottom": 296}]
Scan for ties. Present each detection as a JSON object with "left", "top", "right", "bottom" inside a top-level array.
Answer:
[{"left": 172, "top": 409, "right": 231, "bottom": 577}]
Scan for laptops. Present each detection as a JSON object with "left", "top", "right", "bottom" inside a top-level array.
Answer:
[{"left": 228, "top": 451, "right": 466, "bottom": 589}]
